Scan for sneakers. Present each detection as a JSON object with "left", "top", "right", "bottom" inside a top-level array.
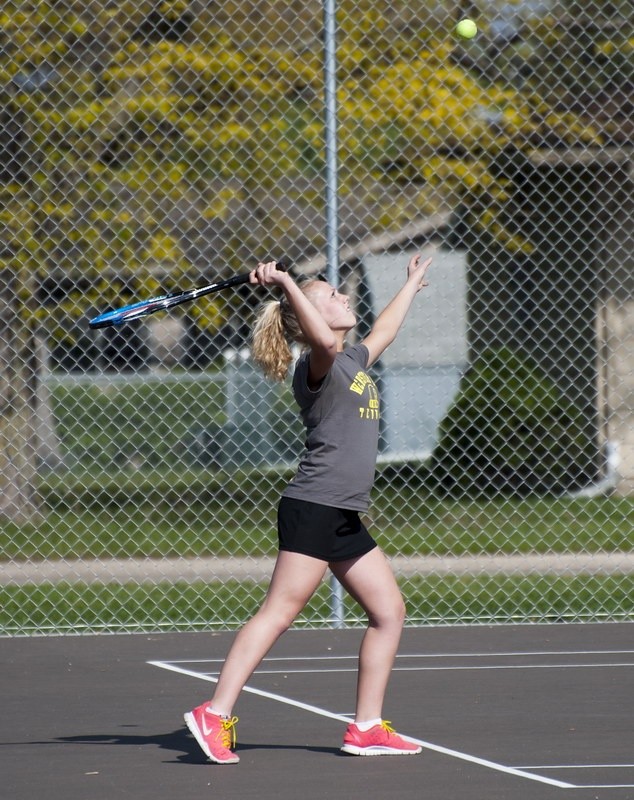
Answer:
[
  {"left": 340, "top": 721, "right": 422, "bottom": 755},
  {"left": 183, "top": 700, "right": 241, "bottom": 763}
]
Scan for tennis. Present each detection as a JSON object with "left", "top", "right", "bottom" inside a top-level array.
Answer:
[{"left": 456, "top": 19, "right": 478, "bottom": 40}]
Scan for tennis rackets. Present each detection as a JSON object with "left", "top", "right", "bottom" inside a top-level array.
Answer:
[{"left": 88, "top": 261, "right": 287, "bottom": 330}]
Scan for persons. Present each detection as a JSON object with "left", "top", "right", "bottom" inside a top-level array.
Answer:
[{"left": 180, "top": 251, "right": 436, "bottom": 763}]
